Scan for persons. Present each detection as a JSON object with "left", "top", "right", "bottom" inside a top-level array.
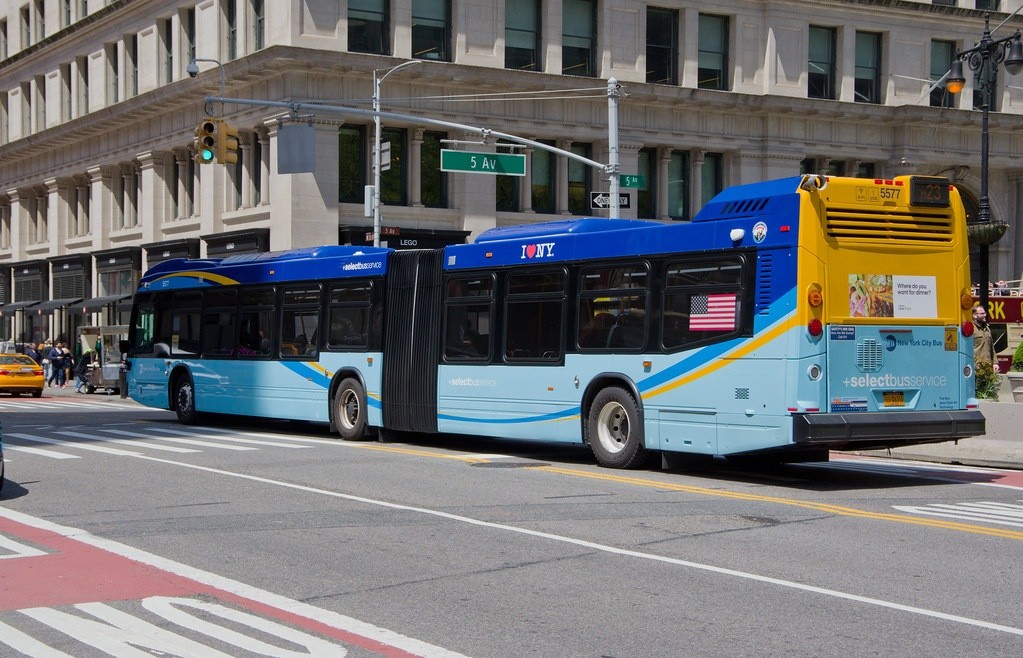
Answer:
[
  {"left": 971, "top": 305, "right": 999, "bottom": 372},
  {"left": 295, "top": 327, "right": 318, "bottom": 355},
  {"left": 334, "top": 312, "right": 354, "bottom": 348},
  {"left": 971, "top": 279, "right": 1006, "bottom": 297},
  {"left": 24, "top": 338, "right": 98, "bottom": 393},
  {"left": 105, "top": 339, "right": 121, "bottom": 362}
]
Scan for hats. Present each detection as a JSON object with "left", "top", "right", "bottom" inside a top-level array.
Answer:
[
  {"left": 44, "top": 340, "right": 53, "bottom": 344},
  {"left": 56, "top": 343, "right": 62, "bottom": 346}
]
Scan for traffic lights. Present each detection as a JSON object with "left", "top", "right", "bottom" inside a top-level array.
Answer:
[
  {"left": 217, "top": 122, "right": 237, "bottom": 164},
  {"left": 198, "top": 118, "right": 216, "bottom": 164}
]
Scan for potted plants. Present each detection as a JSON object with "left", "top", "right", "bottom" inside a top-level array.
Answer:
[{"left": 1005, "top": 340, "right": 1023, "bottom": 403}]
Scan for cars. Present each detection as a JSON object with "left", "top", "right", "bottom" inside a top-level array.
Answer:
[{"left": 0, "top": 353, "right": 45, "bottom": 398}]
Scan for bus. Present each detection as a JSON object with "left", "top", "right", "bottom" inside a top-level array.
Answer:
[{"left": 123, "top": 173, "right": 987, "bottom": 470}]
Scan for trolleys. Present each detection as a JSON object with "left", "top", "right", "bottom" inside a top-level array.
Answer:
[{"left": 77, "top": 326, "right": 149, "bottom": 394}]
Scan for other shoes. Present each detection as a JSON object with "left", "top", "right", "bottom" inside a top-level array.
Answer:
[
  {"left": 76, "top": 390, "right": 82, "bottom": 393},
  {"left": 48, "top": 384, "right": 51, "bottom": 389},
  {"left": 61, "top": 387, "right": 65, "bottom": 391},
  {"left": 54, "top": 384, "right": 58, "bottom": 388}
]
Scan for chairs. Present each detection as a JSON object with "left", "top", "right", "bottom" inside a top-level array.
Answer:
[{"left": 209, "top": 313, "right": 643, "bottom": 360}]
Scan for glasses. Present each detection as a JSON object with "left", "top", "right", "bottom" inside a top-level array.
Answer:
[{"left": 974, "top": 312, "right": 987, "bottom": 314}]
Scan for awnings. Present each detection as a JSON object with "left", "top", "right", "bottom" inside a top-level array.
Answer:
[{"left": 0, "top": 294, "right": 133, "bottom": 316}]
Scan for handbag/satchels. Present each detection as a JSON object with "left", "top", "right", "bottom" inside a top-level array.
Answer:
[{"left": 42, "top": 359, "right": 50, "bottom": 364}]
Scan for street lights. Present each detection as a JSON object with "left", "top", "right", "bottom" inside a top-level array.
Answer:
[
  {"left": 373, "top": 61, "right": 422, "bottom": 248},
  {"left": 945, "top": 12, "right": 1023, "bottom": 330}
]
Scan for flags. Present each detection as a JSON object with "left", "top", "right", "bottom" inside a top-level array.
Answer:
[{"left": 689, "top": 295, "right": 736, "bottom": 330}]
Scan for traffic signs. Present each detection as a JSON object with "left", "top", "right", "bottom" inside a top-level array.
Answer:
[{"left": 590, "top": 191, "right": 631, "bottom": 209}]
